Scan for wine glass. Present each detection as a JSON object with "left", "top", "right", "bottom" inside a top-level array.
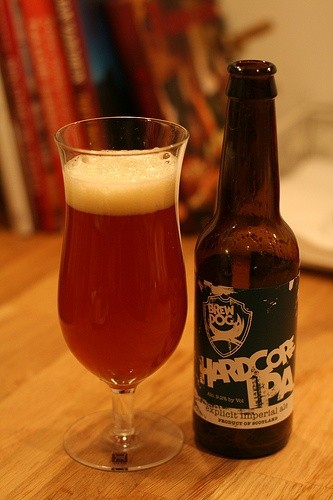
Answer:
[{"left": 53, "top": 116, "right": 184, "bottom": 472}]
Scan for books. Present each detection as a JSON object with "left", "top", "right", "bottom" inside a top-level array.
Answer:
[{"left": 1, "top": 0, "right": 235, "bottom": 236}]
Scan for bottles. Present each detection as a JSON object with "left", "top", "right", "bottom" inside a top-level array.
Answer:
[{"left": 191, "top": 60, "right": 300, "bottom": 459}]
